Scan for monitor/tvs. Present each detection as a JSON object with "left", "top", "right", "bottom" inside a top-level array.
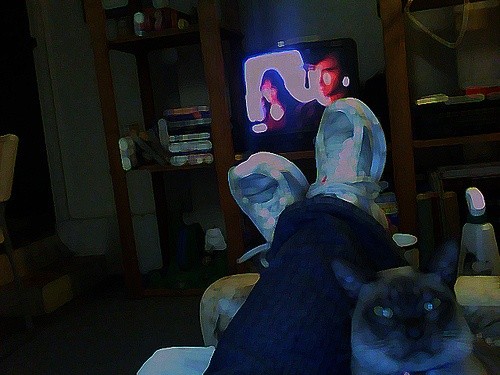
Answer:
[{"left": 237, "top": 37, "right": 362, "bottom": 153}]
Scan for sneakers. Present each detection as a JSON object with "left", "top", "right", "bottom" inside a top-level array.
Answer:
[
  {"left": 228, "top": 153, "right": 310, "bottom": 264},
  {"left": 307, "top": 99, "right": 389, "bottom": 231}
]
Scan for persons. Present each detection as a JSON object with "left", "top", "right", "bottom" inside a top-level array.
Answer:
[
  {"left": 293, "top": 43, "right": 352, "bottom": 116},
  {"left": 262, "top": 69, "right": 305, "bottom": 130},
  {"left": 199, "top": 95, "right": 394, "bottom": 375}
]
